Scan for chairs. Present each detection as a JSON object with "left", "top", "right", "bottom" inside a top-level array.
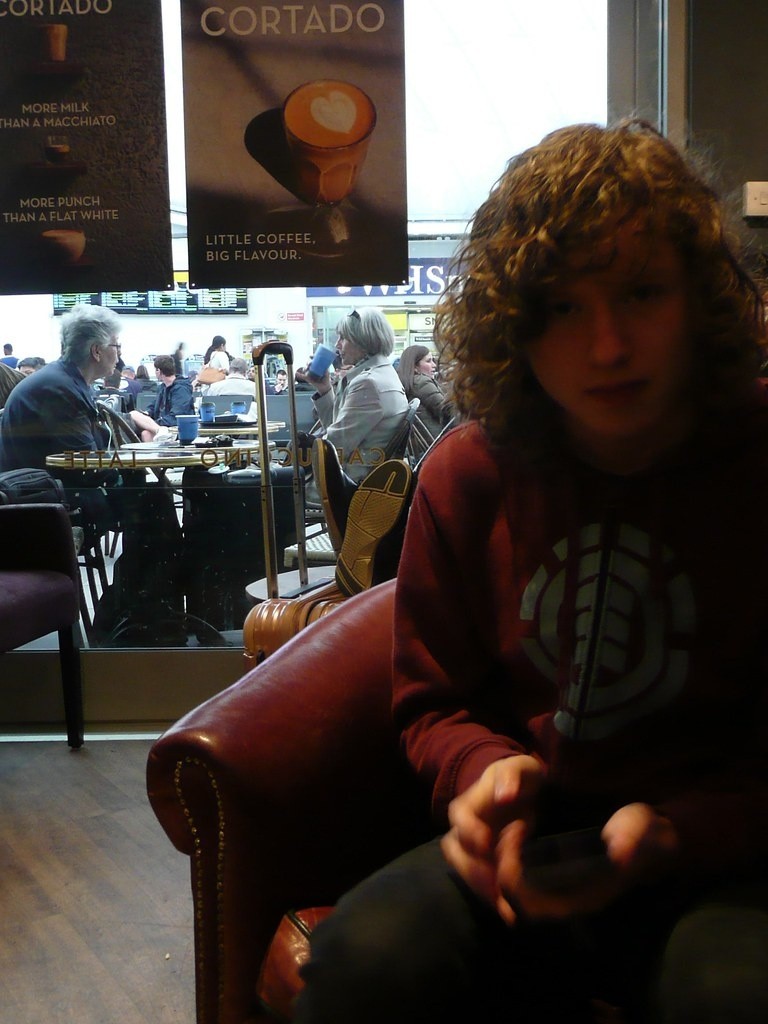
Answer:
[
  {"left": 0, "top": 503, "right": 85, "bottom": 749},
  {"left": 304, "top": 397, "right": 421, "bottom": 525},
  {"left": 96, "top": 400, "right": 184, "bottom": 558},
  {"left": 409, "top": 414, "right": 435, "bottom": 465}
]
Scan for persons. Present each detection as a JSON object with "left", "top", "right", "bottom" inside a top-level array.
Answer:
[
  {"left": 0, "top": 335, "right": 355, "bottom": 444},
  {"left": 306, "top": 306, "right": 408, "bottom": 507},
  {"left": 399, "top": 346, "right": 464, "bottom": 462},
  {"left": 293, "top": 115, "right": 768, "bottom": 1024},
  {"left": 0, "top": 318, "right": 216, "bottom": 628}
]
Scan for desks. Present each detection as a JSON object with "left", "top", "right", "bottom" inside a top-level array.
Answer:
[{"left": 45, "top": 420, "right": 285, "bottom": 468}]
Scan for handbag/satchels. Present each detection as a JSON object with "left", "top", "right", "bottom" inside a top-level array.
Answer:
[{"left": 0, "top": 468, "right": 60, "bottom": 503}]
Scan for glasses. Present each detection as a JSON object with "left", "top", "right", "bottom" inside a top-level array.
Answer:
[
  {"left": 349, "top": 310, "right": 360, "bottom": 321},
  {"left": 108, "top": 344, "right": 121, "bottom": 351}
]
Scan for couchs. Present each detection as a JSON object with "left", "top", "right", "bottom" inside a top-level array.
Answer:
[{"left": 145, "top": 576, "right": 396, "bottom": 1024}]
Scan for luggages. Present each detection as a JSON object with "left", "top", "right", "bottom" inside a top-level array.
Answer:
[
  {"left": 182, "top": 461, "right": 307, "bottom": 630},
  {"left": 242, "top": 340, "right": 351, "bottom": 672}
]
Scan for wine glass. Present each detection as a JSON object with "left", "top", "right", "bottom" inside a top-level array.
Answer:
[{"left": 269, "top": 79, "right": 376, "bottom": 259}]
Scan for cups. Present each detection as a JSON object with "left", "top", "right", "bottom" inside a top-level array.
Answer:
[
  {"left": 175, "top": 414, "right": 199, "bottom": 440},
  {"left": 309, "top": 343, "right": 339, "bottom": 378},
  {"left": 200, "top": 402, "right": 215, "bottom": 422},
  {"left": 41, "top": 229, "right": 87, "bottom": 264},
  {"left": 230, "top": 401, "right": 247, "bottom": 415},
  {"left": 39, "top": 23, "right": 68, "bottom": 62},
  {"left": 46, "top": 135, "right": 70, "bottom": 163}
]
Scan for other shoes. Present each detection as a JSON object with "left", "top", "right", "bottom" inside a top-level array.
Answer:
[
  {"left": 335, "top": 459, "right": 415, "bottom": 596},
  {"left": 313, "top": 437, "right": 359, "bottom": 555}
]
